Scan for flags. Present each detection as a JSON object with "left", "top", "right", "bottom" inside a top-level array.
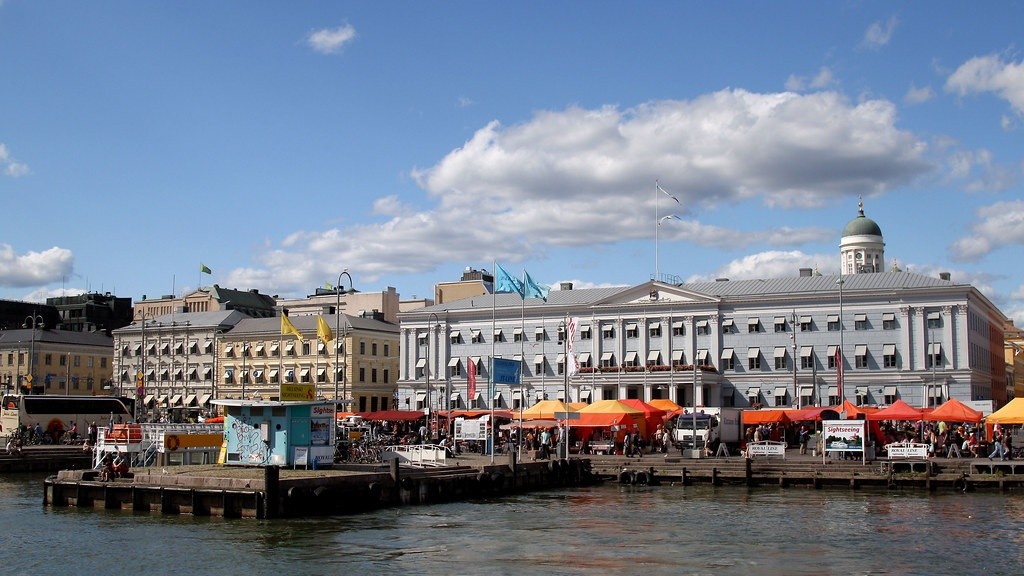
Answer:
[
  {"left": 201, "top": 264, "right": 211, "bottom": 275},
  {"left": 317, "top": 313, "right": 333, "bottom": 347},
  {"left": 282, "top": 312, "right": 304, "bottom": 343},
  {"left": 494, "top": 262, "right": 525, "bottom": 300},
  {"left": 524, "top": 271, "right": 551, "bottom": 302},
  {"left": 326, "top": 282, "right": 332, "bottom": 289},
  {"left": 568, "top": 316, "right": 579, "bottom": 377}
]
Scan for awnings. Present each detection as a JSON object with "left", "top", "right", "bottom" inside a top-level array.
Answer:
[
  {"left": 358, "top": 411, "right": 427, "bottom": 420},
  {"left": 499, "top": 420, "right": 558, "bottom": 431},
  {"left": 437, "top": 410, "right": 513, "bottom": 419}
]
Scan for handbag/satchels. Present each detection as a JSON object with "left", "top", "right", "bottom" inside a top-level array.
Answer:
[
  {"left": 961, "top": 441, "right": 967, "bottom": 449},
  {"left": 929, "top": 443, "right": 934, "bottom": 453}
]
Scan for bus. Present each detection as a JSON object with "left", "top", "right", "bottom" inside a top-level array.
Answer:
[{"left": 0, "top": 394, "right": 138, "bottom": 440}]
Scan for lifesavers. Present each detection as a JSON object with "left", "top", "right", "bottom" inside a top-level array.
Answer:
[
  {"left": 369, "top": 482, "right": 381, "bottom": 500},
  {"left": 167, "top": 434, "right": 180, "bottom": 450},
  {"left": 549, "top": 459, "right": 570, "bottom": 478},
  {"left": 617, "top": 470, "right": 651, "bottom": 486},
  {"left": 402, "top": 476, "right": 414, "bottom": 491},
  {"left": 287, "top": 486, "right": 336, "bottom": 516},
  {"left": 953, "top": 478, "right": 967, "bottom": 492},
  {"left": 476, "top": 472, "right": 505, "bottom": 491}
]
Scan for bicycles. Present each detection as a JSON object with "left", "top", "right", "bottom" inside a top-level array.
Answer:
[
  {"left": 333, "top": 436, "right": 382, "bottom": 464},
  {"left": 9, "top": 428, "right": 83, "bottom": 446}
]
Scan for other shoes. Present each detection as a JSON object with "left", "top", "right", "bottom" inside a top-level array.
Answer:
[
  {"left": 100, "top": 480, "right": 106, "bottom": 482},
  {"left": 1001, "top": 459, "right": 1007, "bottom": 461},
  {"left": 975, "top": 454, "right": 979, "bottom": 458},
  {"left": 120, "top": 474, "right": 124, "bottom": 478},
  {"left": 989, "top": 456, "right": 993, "bottom": 461},
  {"left": 105, "top": 479, "right": 109, "bottom": 482}
]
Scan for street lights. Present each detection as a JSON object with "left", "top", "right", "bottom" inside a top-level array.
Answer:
[
  {"left": 21, "top": 308, "right": 45, "bottom": 394},
  {"left": 835, "top": 268, "right": 846, "bottom": 419},
  {"left": 130, "top": 304, "right": 157, "bottom": 421},
  {"left": 425, "top": 312, "right": 441, "bottom": 431},
  {"left": 209, "top": 329, "right": 226, "bottom": 419},
  {"left": 333, "top": 272, "right": 362, "bottom": 448},
  {"left": 788, "top": 308, "right": 801, "bottom": 398},
  {"left": 556, "top": 319, "right": 569, "bottom": 461}
]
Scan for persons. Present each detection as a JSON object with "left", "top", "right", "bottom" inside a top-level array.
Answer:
[
  {"left": 703, "top": 426, "right": 713, "bottom": 457},
  {"left": 469, "top": 441, "right": 478, "bottom": 453},
  {"left": 746, "top": 424, "right": 809, "bottom": 454},
  {"left": 99, "top": 451, "right": 128, "bottom": 482},
  {"left": 818, "top": 421, "right": 1015, "bottom": 462},
  {"left": 338, "top": 420, "right": 426, "bottom": 444},
  {"left": 18, "top": 422, "right": 42, "bottom": 444},
  {"left": 5, "top": 438, "right": 17, "bottom": 455},
  {"left": 68, "top": 423, "right": 77, "bottom": 439},
  {"left": 623, "top": 423, "right": 669, "bottom": 457},
  {"left": 87, "top": 420, "right": 98, "bottom": 443},
  {"left": 117, "top": 414, "right": 122, "bottom": 423},
  {"left": 438, "top": 434, "right": 453, "bottom": 454},
  {"left": 455, "top": 427, "right": 553, "bottom": 460}
]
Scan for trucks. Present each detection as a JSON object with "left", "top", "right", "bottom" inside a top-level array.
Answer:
[{"left": 673, "top": 407, "right": 741, "bottom": 455}]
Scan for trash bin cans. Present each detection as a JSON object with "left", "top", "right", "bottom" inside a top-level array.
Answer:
[{"left": 557, "top": 442, "right": 566, "bottom": 458}]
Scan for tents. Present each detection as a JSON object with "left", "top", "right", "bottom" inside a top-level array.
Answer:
[
  {"left": 513, "top": 399, "right": 683, "bottom": 454},
  {"left": 742, "top": 400, "right": 865, "bottom": 424},
  {"left": 866, "top": 397, "right": 1024, "bottom": 442}
]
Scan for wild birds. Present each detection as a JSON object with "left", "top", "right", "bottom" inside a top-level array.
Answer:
[{"left": 163, "top": 468, "right": 169, "bottom": 475}]
